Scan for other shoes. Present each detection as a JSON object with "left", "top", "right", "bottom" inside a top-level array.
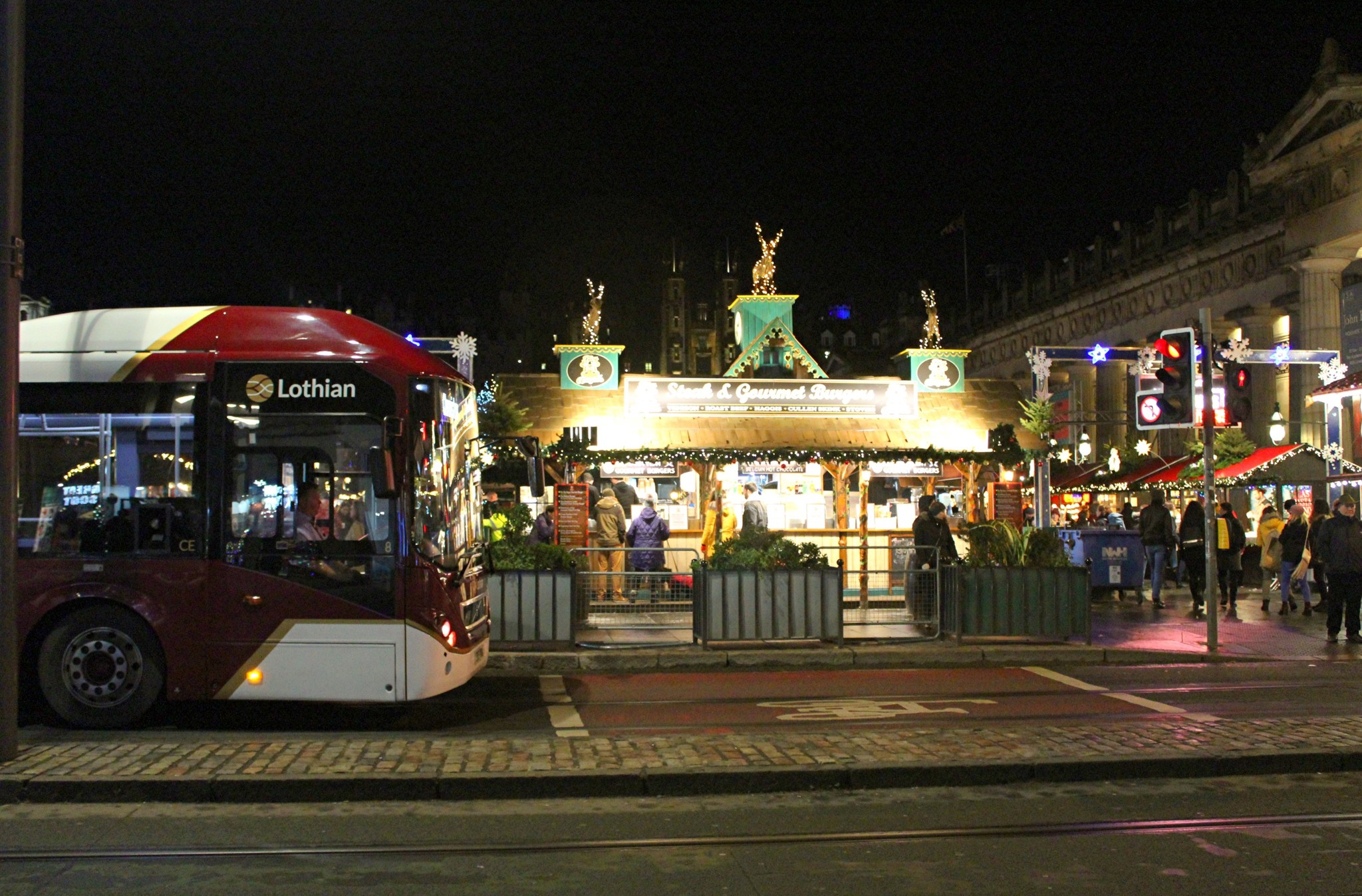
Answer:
[
  {"left": 1278, "top": 604, "right": 1289, "bottom": 615},
  {"left": 612, "top": 593, "right": 629, "bottom": 602},
  {"left": 650, "top": 590, "right": 659, "bottom": 606},
  {"left": 1313, "top": 599, "right": 1326, "bottom": 612},
  {"left": 1261, "top": 599, "right": 1270, "bottom": 611},
  {"left": 1193, "top": 603, "right": 1204, "bottom": 614},
  {"left": 1194, "top": 590, "right": 1204, "bottom": 606},
  {"left": 1230, "top": 601, "right": 1237, "bottom": 610},
  {"left": 1290, "top": 602, "right": 1297, "bottom": 611},
  {"left": 1346, "top": 634, "right": 1362, "bottom": 643},
  {"left": 630, "top": 589, "right": 638, "bottom": 603},
  {"left": 1220, "top": 596, "right": 1228, "bottom": 605},
  {"left": 1302, "top": 602, "right": 1312, "bottom": 616},
  {"left": 1327, "top": 631, "right": 1338, "bottom": 642},
  {"left": 597, "top": 595, "right": 605, "bottom": 602}
]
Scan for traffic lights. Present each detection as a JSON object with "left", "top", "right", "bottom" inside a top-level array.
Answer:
[
  {"left": 1222, "top": 362, "right": 1253, "bottom": 422},
  {"left": 1135, "top": 326, "right": 1195, "bottom": 431}
]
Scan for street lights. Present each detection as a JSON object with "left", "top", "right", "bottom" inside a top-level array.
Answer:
[{"left": 1266, "top": 401, "right": 1287, "bottom": 521}]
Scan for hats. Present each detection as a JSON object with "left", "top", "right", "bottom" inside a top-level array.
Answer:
[
  {"left": 1290, "top": 505, "right": 1304, "bottom": 516},
  {"left": 918, "top": 494, "right": 936, "bottom": 514},
  {"left": 1338, "top": 494, "right": 1360, "bottom": 505},
  {"left": 1284, "top": 499, "right": 1296, "bottom": 509},
  {"left": 1333, "top": 498, "right": 1340, "bottom": 511},
  {"left": 929, "top": 502, "right": 946, "bottom": 516}
]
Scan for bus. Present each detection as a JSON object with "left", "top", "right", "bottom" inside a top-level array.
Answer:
[{"left": 19, "top": 302, "right": 546, "bottom": 733}]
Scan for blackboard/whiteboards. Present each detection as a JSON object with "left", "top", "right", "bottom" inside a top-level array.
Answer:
[{"left": 888, "top": 534, "right": 916, "bottom": 585}]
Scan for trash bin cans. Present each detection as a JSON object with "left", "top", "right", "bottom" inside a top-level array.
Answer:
[{"left": 1057, "top": 529, "right": 1148, "bottom": 604}]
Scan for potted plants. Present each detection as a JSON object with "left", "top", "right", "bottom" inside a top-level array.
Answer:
[
  {"left": 688, "top": 510, "right": 847, "bottom": 645},
  {"left": 482, "top": 503, "right": 584, "bottom": 653},
  {"left": 915, "top": 512, "right": 1099, "bottom": 645}
]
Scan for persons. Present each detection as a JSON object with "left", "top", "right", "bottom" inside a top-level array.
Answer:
[
  {"left": 1021, "top": 486, "right": 1362, "bottom": 644},
  {"left": 703, "top": 490, "right": 740, "bottom": 560},
  {"left": 908, "top": 494, "right": 960, "bottom": 633},
  {"left": 480, "top": 473, "right": 669, "bottom": 605},
  {"left": 290, "top": 481, "right": 332, "bottom": 543},
  {"left": 737, "top": 483, "right": 769, "bottom": 542}
]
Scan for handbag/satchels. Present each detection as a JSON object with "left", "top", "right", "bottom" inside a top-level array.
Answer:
[
  {"left": 1229, "top": 553, "right": 1243, "bottom": 572},
  {"left": 1259, "top": 529, "right": 1283, "bottom": 573},
  {"left": 914, "top": 558, "right": 937, "bottom": 594},
  {"left": 1298, "top": 551, "right": 1325, "bottom": 569}
]
